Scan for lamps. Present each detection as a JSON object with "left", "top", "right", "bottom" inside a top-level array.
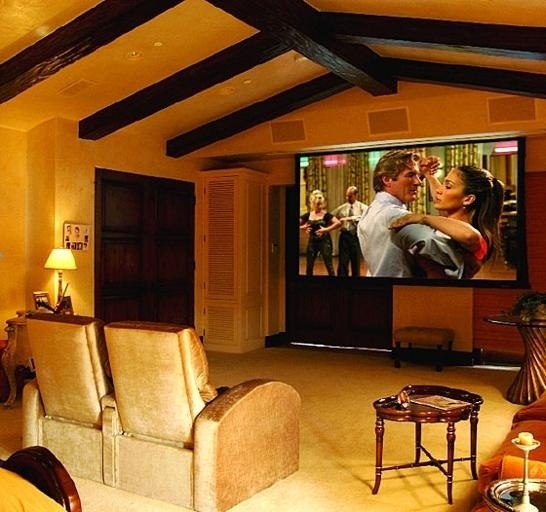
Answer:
[{"left": 44, "top": 246, "right": 77, "bottom": 309}]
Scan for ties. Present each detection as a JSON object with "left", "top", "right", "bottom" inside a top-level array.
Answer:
[{"left": 349, "top": 206, "right": 354, "bottom": 216}]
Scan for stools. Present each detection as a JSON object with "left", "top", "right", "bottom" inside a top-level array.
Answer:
[{"left": 394, "top": 328, "right": 453, "bottom": 371}]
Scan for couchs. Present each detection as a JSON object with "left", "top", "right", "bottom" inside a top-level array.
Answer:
[
  {"left": 23, "top": 316, "right": 113, "bottom": 488},
  {"left": 104, "top": 321, "right": 302, "bottom": 510}
]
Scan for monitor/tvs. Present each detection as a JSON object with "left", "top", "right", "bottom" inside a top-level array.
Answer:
[{"left": 295, "top": 136, "right": 531, "bottom": 290}]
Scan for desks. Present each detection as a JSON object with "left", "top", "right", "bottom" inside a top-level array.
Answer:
[{"left": 0, "top": 318, "right": 34, "bottom": 405}]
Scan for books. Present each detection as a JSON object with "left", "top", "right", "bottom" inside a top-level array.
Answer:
[{"left": 410, "top": 394, "right": 472, "bottom": 412}]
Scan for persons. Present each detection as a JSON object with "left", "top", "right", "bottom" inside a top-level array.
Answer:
[
  {"left": 327, "top": 184, "right": 370, "bottom": 276},
  {"left": 355, "top": 148, "right": 481, "bottom": 279},
  {"left": 386, "top": 154, "right": 505, "bottom": 279},
  {"left": 300, "top": 188, "right": 343, "bottom": 276},
  {"left": 64, "top": 224, "right": 90, "bottom": 250}
]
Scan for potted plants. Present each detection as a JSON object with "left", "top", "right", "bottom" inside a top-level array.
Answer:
[{"left": 500, "top": 291, "right": 546, "bottom": 321}]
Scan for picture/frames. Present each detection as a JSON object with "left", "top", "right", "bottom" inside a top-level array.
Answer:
[
  {"left": 34, "top": 292, "right": 51, "bottom": 311},
  {"left": 59, "top": 296, "right": 74, "bottom": 314}
]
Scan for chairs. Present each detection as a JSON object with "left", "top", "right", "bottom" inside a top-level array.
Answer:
[
  {"left": 475, "top": 385, "right": 546, "bottom": 512},
  {"left": 7, "top": 445, "right": 82, "bottom": 512}
]
